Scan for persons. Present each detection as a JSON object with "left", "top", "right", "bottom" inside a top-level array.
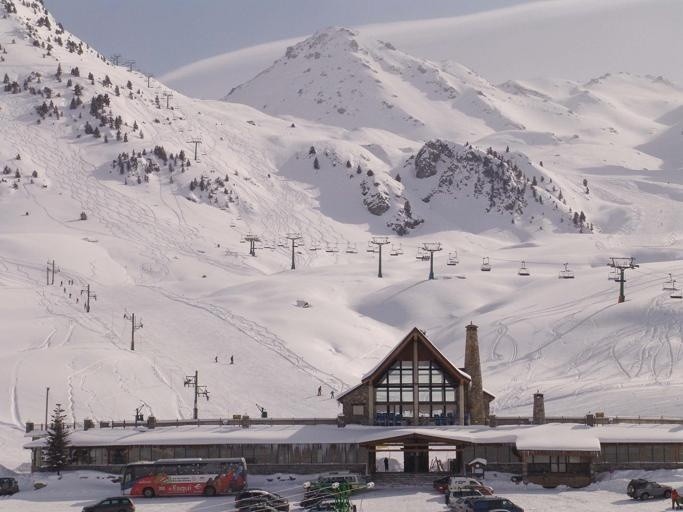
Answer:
[
  {"left": 214, "top": 356, "right": 218, "bottom": 362},
  {"left": 317, "top": 385, "right": 321, "bottom": 396},
  {"left": 330, "top": 391, "right": 335, "bottom": 399},
  {"left": 384, "top": 458, "right": 388, "bottom": 472},
  {"left": 671, "top": 488, "right": 681, "bottom": 509},
  {"left": 230, "top": 355, "right": 234, "bottom": 364}
]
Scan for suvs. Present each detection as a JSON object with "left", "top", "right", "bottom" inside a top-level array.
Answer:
[{"left": 625, "top": 477, "right": 673, "bottom": 501}]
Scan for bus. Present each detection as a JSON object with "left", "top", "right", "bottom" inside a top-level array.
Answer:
[{"left": 117, "top": 455, "right": 251, "bottom": 498}]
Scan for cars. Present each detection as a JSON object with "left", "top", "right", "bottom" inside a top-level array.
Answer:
[
  {"left": 78, "top": 495, "right": 136, "bottom": 512},
  {"left": 230, "top": 471, "right": 366, "bottom": 512},
  {"left": 433, "top": 475, "right": 525, "bottom": 512},
  {"left": 0, "top": 476, "right": 19, "bottom": 496}
]
